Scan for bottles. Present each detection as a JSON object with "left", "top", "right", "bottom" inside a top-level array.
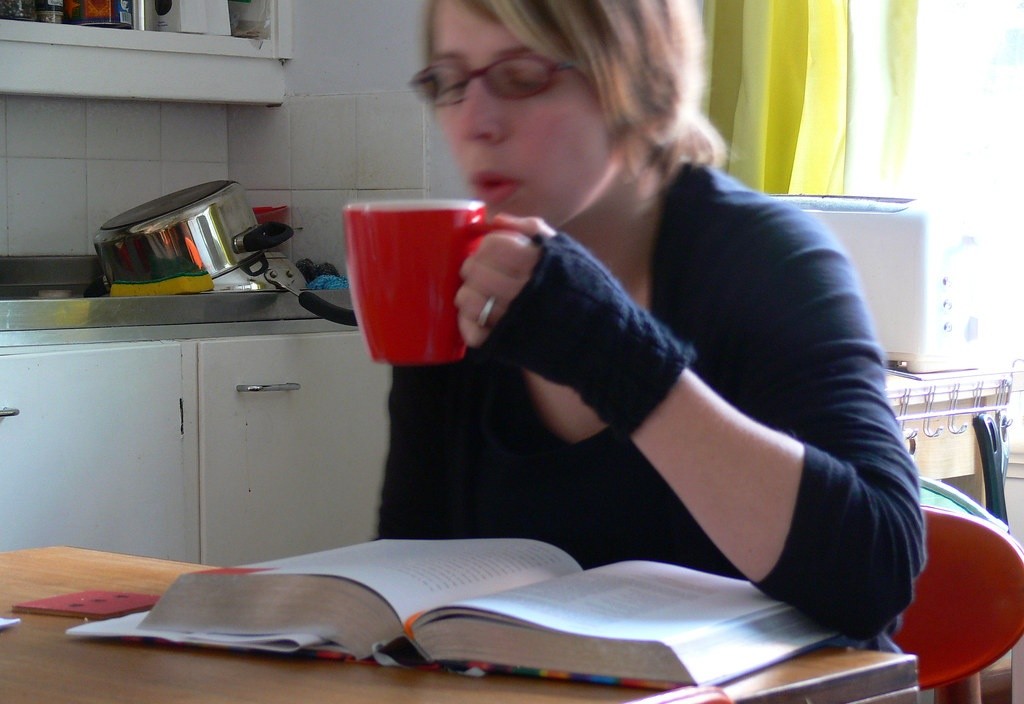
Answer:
[{"left": 0, "top": 0, "right": 133, "bottom": 29}]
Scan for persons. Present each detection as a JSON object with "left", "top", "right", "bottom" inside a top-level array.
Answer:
[{"left": 371, "top": 1, "right": 929, "bottom": 656}]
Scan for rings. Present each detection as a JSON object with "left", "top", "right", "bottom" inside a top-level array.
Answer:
[{"left": 476, "top": 295, "right": 497, "bottom": 328}]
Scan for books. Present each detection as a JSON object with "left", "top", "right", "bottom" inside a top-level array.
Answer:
[{"left": 65, "top": 538, "right": 842, "bottom": 689}]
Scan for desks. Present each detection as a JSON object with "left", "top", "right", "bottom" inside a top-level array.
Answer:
[
  {"left": 881, "top": 373, "right": 1024, "bottom": 704},
  {"left": 0, "top": 548, "right": 920, "bottom": 704}
]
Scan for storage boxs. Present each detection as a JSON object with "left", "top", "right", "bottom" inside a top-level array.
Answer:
[{"left": 145, "top": 0, "right": 231, "bottom": 35}]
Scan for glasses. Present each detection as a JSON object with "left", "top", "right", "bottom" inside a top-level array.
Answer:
[{"left": 409, "top": 52, "right": 582, "bottom": 108}]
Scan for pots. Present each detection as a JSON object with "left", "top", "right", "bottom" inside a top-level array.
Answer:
[
  {"left": 212, "top": 253, "right": 357, "bottom": 328},
  {"left": 95, "top": 179, "right": 295, "bottom": 287}
]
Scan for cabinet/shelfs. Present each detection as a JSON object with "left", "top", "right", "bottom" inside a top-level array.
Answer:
[
  {"left": 0, "top": 0, "right": 294, "bottom": 105},
  {"left": 1, "top": 319, "right": 393, "bottom": 567}
]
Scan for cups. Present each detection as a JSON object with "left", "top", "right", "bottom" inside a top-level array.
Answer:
[{"left": 343, "top": 199, "right": 500, "bottom": 365}]
